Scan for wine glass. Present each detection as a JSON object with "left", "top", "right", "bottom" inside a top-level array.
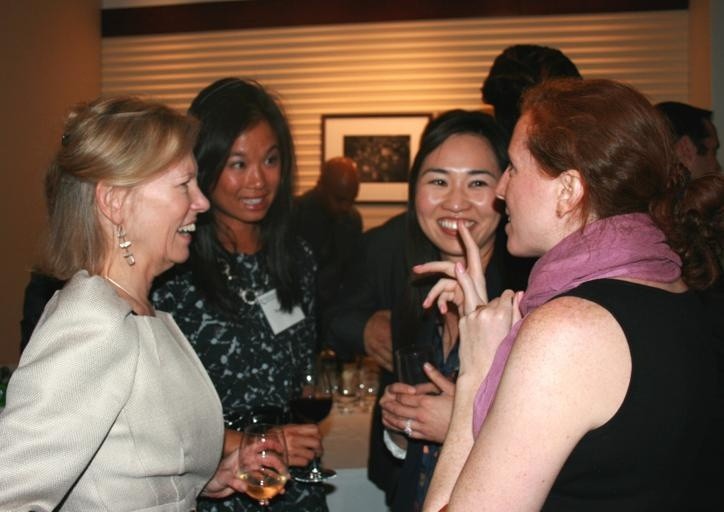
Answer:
[{"left": 237, "top": 369, "right": 336, "bottom": 512}]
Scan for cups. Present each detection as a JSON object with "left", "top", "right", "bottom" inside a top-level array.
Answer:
[
  {"left": 340, "top": 364, "right": 381, "bottom": 416},
  {"left": 394, "top": 345, "right": 430, "bottom": 389}
]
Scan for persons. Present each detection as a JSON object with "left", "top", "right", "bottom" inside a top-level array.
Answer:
[
  {"left": 329, "top": 43, "right": 724, "bottom": 511},
  {"left": 294, "top": 156, "right": 363, "bottom": 361},
  {"left": 0, "top": 92, "right": 291, "bottom": 512},
  {"left": 149, "top": 77, "right": 330, "bottom": 512}
]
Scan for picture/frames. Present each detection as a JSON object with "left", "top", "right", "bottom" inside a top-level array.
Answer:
[{"left": 320, "top": 112, "right": 433, "bottom": 207}]
